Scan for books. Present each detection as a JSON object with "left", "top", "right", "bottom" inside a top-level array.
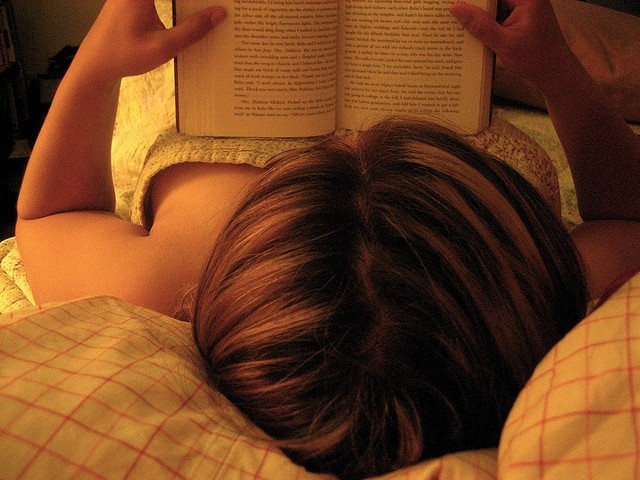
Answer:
[{"left": 172, "top": 1, "right": 498, "bottom": 139}]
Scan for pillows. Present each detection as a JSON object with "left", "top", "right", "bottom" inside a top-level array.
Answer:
[{"left": 2, "top": 268, "right": 640, "bottom": 478}]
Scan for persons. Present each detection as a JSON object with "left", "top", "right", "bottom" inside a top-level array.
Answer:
[{"left": 14, "top": 0, "right": 640, "bottom": 480}]
